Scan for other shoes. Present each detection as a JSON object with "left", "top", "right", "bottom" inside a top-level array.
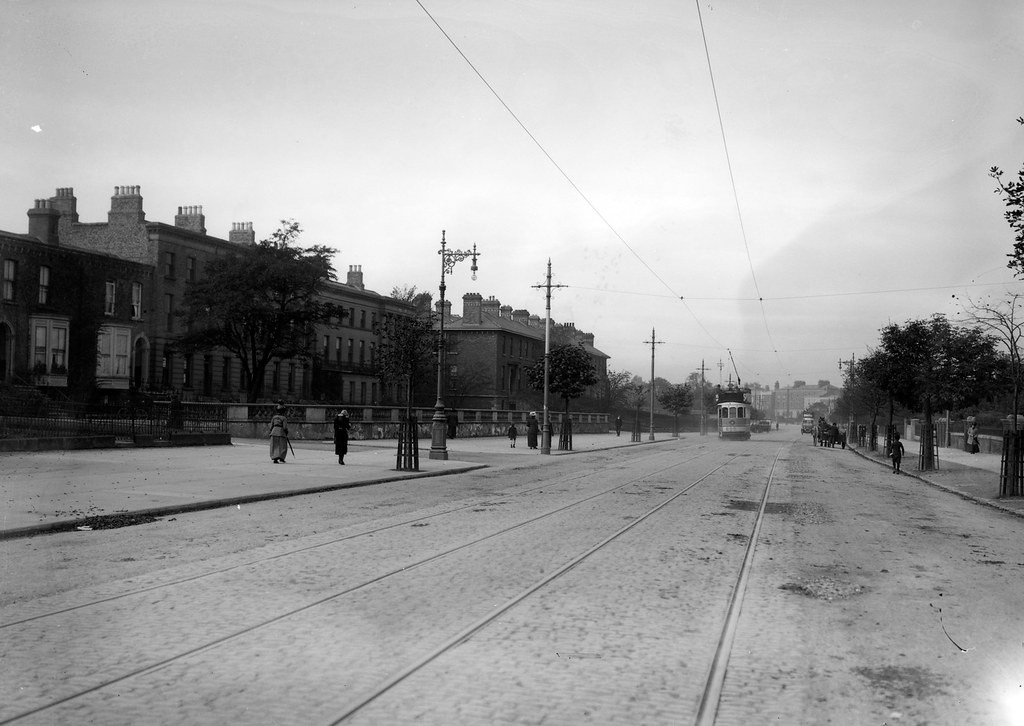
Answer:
[
  {"left": 534, "top": 447, "right": 537, "bottom": 449},
  {"left": 529, "top": 447, "right": 532, "bottom": 449},
  {"left": 280, "top": 459, "right": 286, "bottom": 463},
  {"left": 338, "top": 461, "right": 344, "bottom": 465},
  {"left": 274, "top": 461, "right": 279, "bottom": 463},
  {"left": 511, "top": 444, "right": 512, "bottom": 448},
  {"left": 893, "top": 471, "right": 895, "bottom": 474},
  {"left": 896, "top": 472, "right": 899, "bottom": 474},
  {"left": 513, "top": 444, "right": 515, "bottom": 448}
]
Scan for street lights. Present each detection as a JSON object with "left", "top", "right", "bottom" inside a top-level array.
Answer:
[{"left": 429, "top": 228, "right": 482, "bottom": 456}]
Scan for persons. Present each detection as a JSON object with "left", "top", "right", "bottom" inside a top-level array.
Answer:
[
  {"left": 615, "top": 416, "right": 622, "bottom": 437},
  {"left": 889, "top": 432, "right": 905, "bottom": 475},
  {"left": 447, "top": 410, "right": 458, "bottom": 439},
  {"left": 268, "top": 405, "right": 289, "bottom": 463},
  {"left": 967, "top": 424, "right": 978, "bottom": 454},
  {"left": 541, "top": 416, "right": 554, "bottom": 449},
  {"left": 827, "top": 423, "right": 839, "bottom": 448},
  {"left": 837, "top": 426, "right": 845, "bottom": 445},
  {"left": 333, "top": 410, "right": 351, "bottom": 465},
  {"left": 526, "top": 411, "right": 538, "bottom": 449},
  {"left": 508, "top": 423, "right": 517, "bottom": 449}
]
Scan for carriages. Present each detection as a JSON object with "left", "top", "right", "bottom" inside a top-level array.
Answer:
[{"left": 814, "top": 416, "right": 847, "bottom": 450}]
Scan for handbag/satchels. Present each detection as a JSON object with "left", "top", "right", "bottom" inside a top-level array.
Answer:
[{"left": 890, "top": 453, "right": 893, "bottom": 457}]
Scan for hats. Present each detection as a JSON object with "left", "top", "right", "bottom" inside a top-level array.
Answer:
[
  {"left": 338, "top": 410, "right": 350, "bottom": 418},
  {"left": 529, "top": 411, "right": 536, "bottom": 416}
]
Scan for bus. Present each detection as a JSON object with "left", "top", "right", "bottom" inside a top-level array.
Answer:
[
  {"left": 715, "top": 379, "right": 755, "bottom": 440},
  {"left": 801, "top": 408, "right": 816, "bottom": 435}
]
